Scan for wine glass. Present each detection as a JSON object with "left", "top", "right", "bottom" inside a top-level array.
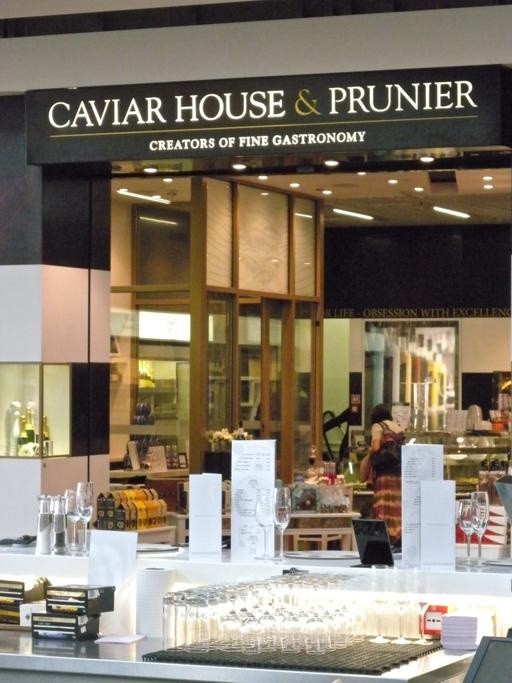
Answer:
[
  {"left": 38, "top": 483, "right": 92, "bottom": 553},
  {"left": 373, "top": 592, "right": 435, "bottom": 646},
  {"left": 158, "top": 570, "right": 364, "bottom": 653},
  {"left": 258, "top": 484, "right": 289, "bottom": 562},
  {"left": 460, "top": 489, "right": 488, "bottom": 566}
]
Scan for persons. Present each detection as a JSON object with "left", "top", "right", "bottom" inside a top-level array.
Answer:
[{"left": 364, "top": 405, "right": 406, "bottom": 547}]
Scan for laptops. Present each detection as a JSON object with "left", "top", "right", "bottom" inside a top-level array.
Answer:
[{"left": 353, "top": 518, "right": 396, "bottom": 567}]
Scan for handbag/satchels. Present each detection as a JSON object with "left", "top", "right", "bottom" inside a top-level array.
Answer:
[{"left": 371, "top": 441, "right": 401, "bottom": 471}]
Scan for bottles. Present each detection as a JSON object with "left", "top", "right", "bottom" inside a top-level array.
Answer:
[
  {"left": 367, "top": 319, "right": 445, "bottom": 416},
  {"left": 17, "top": 405, "right": 51, "bottom": 457}
]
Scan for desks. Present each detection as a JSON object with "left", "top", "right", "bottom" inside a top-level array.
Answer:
[{"left": 166, "top": 508, "right": 361, "bottom": 555}]
